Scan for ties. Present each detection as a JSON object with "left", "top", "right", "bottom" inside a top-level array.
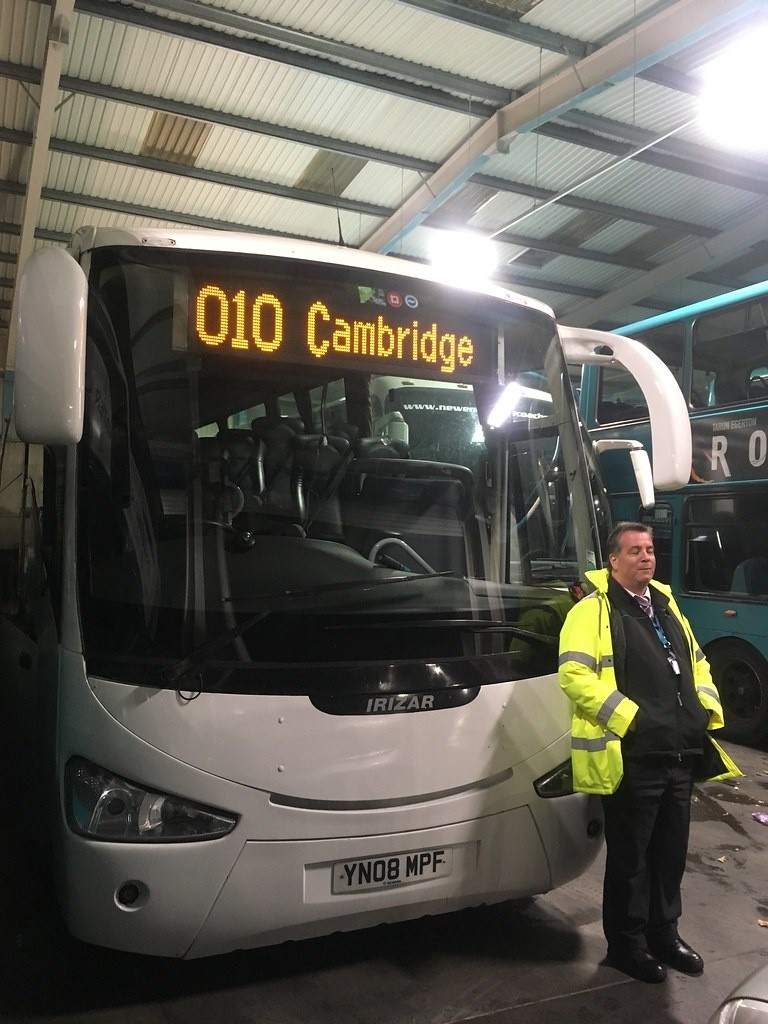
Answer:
[{"left": 634, "top": 595, "right": 653, "bottom": 619}]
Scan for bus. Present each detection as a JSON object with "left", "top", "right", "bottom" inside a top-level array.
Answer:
[
  {"left": 577, "top": 276, "right": 768, "bottom": 743},
  {"left": 12, "top": 224, "right": 693, "bottom": 957},
  {"left": 294, "top": 376, "right": 555, "bottom": 505}
]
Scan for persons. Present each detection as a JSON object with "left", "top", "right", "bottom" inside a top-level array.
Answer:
[
  {"left": 507, "top": 548, "right": 577, "bottom": 681},
  {"left": 557, "top": 520, "right": 746, "bottom": 984}
]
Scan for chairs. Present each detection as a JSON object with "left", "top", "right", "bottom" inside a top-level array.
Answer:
[
  {"left": 713, "top": 366, "right": 768, "bottom": 404},
  {"left": 138, "top": 416, "right": 495, "bottom": 580},
  {"left": 599, "top": 402, "right": 649, "bottom": 425}
]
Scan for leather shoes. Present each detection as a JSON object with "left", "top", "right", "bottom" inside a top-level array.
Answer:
[
  {"left": 647, "top": 932, "right": 704, "bottom": 974},
  {"left": 607, "top": 942, "right": 667, "bottom": 984}
]
